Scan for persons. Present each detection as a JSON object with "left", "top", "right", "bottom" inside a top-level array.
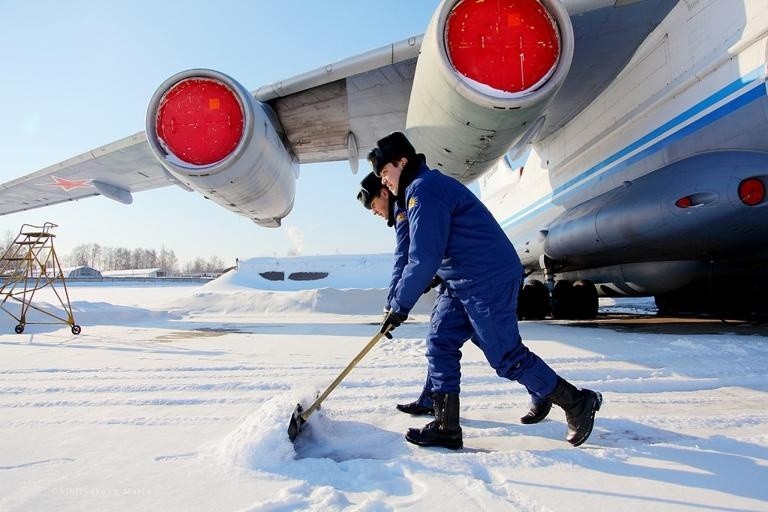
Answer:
[
  {"left": 356, "top": 170, "right": 551, "bottom": 425},
  {"left": 368, "top": 130, "right": 603, "bottom": 451}
]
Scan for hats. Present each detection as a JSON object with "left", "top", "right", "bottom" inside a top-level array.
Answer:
[
  {"left": 357, "top": 172, "right": 386, "bottom": 209},
  {"left": 367, "top": 132, "right": 415, "bottom": 177}
]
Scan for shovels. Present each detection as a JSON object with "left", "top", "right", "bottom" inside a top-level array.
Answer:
[{"left": 286, "top": 313, "right": 402, "bottom": 443}]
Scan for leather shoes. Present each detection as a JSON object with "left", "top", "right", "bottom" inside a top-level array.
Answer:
[
  {"left": 520, "top": 399, "right": 552, "bottom": 424},
  {"left": 397, "top": 401, "right": 435, "bottom": 416}
]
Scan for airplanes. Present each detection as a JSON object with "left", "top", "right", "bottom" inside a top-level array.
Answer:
[{"left": 0, "top": 2, "right": 768, "bottom": 328}]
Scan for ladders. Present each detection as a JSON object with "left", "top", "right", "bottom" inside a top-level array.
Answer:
[{"left": 0, "top": 221, "right": 81, "bottom": 335}]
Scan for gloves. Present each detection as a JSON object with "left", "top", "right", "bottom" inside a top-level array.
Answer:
[{"left": 380, "top": 307, "right": 407, "bottom": 339}]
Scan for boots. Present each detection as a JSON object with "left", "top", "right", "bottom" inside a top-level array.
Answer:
[
  {"left": 404, "top": 392, "right": 462, "bottom": 450},
  {"left": 547, "top": 375, "right": 602, "bottom": 446}
]
